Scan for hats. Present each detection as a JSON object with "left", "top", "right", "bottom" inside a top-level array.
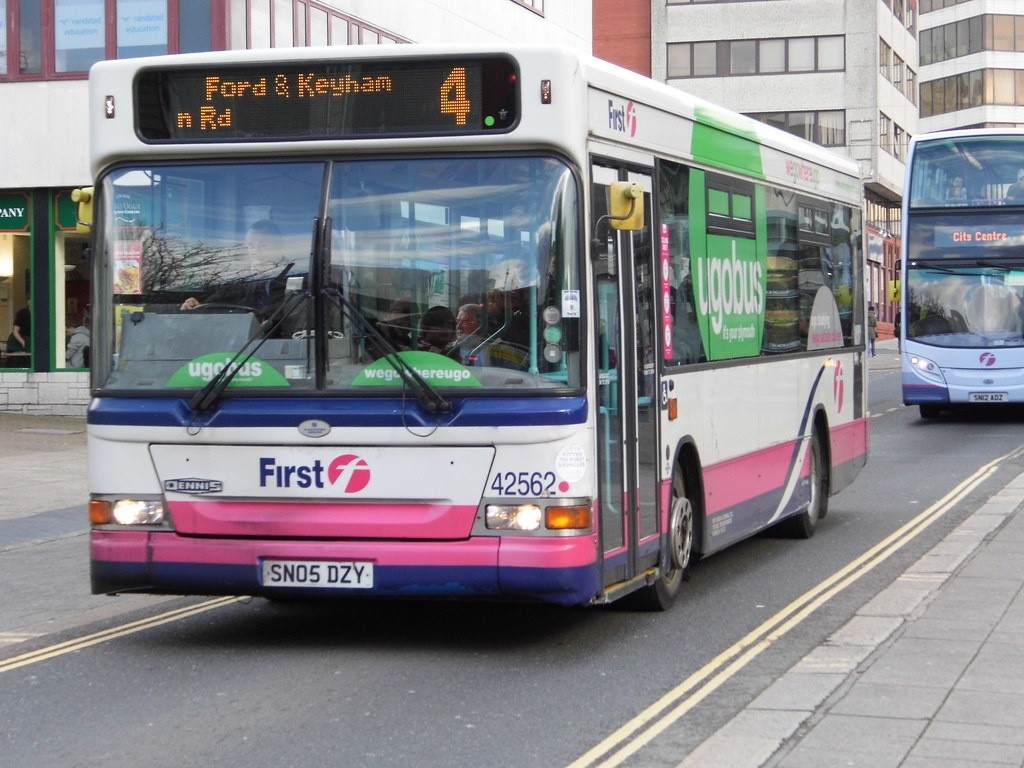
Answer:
[{"left": 1017, "top": 169, "right": 1024, "bottom": 181}]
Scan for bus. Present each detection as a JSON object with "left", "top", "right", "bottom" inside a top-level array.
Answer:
[
  {"left": 885, "top": 126, "right": 1024, "bottom": 419},
  {"left": 67, "top": 40, "right": 872, "bottom": 614}
]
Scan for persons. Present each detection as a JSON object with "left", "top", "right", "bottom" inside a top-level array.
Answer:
[
  {"left": 868, "top": 307, "right": 878, "bottom": 356},
  {"left": 65, "top": 304, "right": 92, "bottom": 368},
  {"left": 894, "top": 306, "right": 901, "bottom": 354},
  {"left": 180, "top": 219, "right": 310, "bottom": 330},
  {"left": 365, "top": 269, "right": 535, "bottom": 369},
  {"left": 5, "top": 300, "right": 31, "bottom": 368}
]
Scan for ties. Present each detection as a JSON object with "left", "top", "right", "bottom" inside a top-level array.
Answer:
[{"left": 257, "top": 277, "right": 267, "bottom": 299}]
